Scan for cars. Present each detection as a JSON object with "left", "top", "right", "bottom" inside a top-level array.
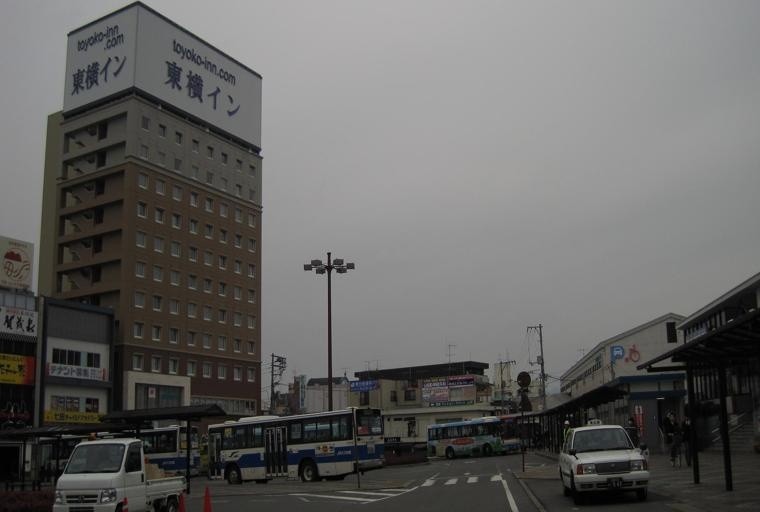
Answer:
[{"left": 558, "top": 422, "right": 650, "bottom": 503}]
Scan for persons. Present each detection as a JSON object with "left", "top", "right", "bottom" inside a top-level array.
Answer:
[
  {"left": 662, "top": 410, "right": 702, "bottom": 467},
  {"left": 563, "top": 420, "right": 571, "bottom": 441},
  {"left": 626, "top": 417, "right": 638, "bottom": 446}
]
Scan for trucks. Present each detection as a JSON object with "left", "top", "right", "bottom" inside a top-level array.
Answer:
[{"left": 52, "top": 437, "right": 186, "bottom": 512}]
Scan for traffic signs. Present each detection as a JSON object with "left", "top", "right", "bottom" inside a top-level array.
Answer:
[{"left": 350, "top": 380, "right": 378, "bottom": 393}]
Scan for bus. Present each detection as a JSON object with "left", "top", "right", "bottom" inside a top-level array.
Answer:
[
  {"left": 36, "top": 426, "right": 201, "bottom": 478},
  {"left": 207, "top": 406, "right": 386, "bottom": 483},
  {"left": 425, "top": 416, "right": 520, "bottom": 460}
]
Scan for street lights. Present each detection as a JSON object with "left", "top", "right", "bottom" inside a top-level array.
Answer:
[{"left": 303, "top": 253, "right": 355, "bottom": 410}]
